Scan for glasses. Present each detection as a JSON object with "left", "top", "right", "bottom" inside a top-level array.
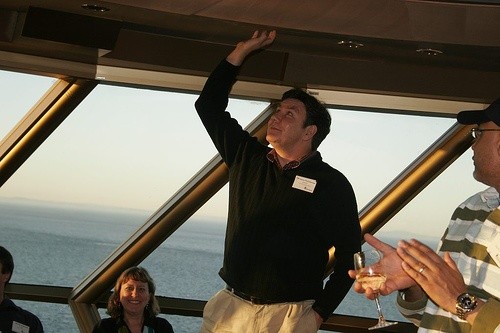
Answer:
[{"left": 471, "top": 128, "right": 500, "bottom": 139}]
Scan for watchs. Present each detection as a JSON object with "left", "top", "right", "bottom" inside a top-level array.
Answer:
[{"left": 456, "top": 292, "right": 479, "bottom": 320}]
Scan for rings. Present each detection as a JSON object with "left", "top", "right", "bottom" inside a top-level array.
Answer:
[{"left": 419, "top": 266, "right": 426, "bottom": 273}]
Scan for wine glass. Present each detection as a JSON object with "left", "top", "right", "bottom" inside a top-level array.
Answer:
[{"left": 354, "top": 249, "right": 398, "bottom": 330}]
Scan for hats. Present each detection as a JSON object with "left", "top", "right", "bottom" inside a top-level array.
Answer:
[{"left": 457, "top": 99, "right": 500, "bottom": 127}]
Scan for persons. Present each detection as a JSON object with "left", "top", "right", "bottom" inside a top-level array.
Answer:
[
  {"left": 92, "top": 267, "right": 174, "bottom": 333},
  {"left": 195, "top": 30, "right": 365, "bottom": 333},
  {"left": 0, "top": 246, "right": 44, "bottom": 333},
  {"left": 348, "top": 100, "right": 500, "bottom": 333}
]
